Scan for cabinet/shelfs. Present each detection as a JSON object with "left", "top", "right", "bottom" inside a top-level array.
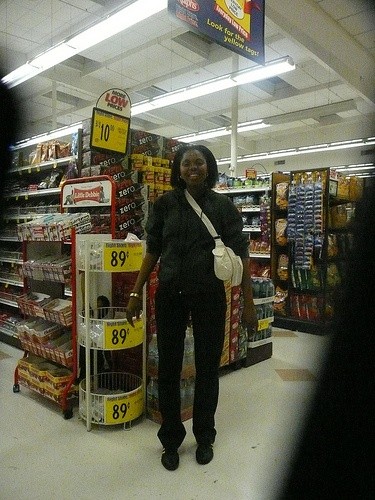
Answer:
[
  {"left": 13, "top": 227, "right": 77, "bottom": 419},
  {"left": 231, "top": 296, "right": 274, "bottom": 371},
  {"left": 0, "top": 129, "right": 119, "bottom": 370},
  {"left": 211, "top": 173, "right": 361, "bottom": 337}
]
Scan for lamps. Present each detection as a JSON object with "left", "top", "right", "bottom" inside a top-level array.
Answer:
[
  {"left": 216, "top": 101, "right": 375, "bottom": 166},
  {"left": 9, "top": 55, "right": 296, "bottom": 151},
  {"left": 0, "top": 0, "right": 169, "bottom": 90},
  {"left": 170, "top": 53, "right": 358, "bottom": 145}
]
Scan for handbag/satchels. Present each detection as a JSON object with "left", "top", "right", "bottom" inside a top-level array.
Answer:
[{"left": 183, "top": 188, "right": 243, "bottom": 287}]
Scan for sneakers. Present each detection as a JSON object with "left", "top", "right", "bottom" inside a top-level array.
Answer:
[
  {"left": 195, "top": 443, "right": 214, "bottom": 465},
  {"left": 162, "top": 447, "right": 180, "bottom": 471}
]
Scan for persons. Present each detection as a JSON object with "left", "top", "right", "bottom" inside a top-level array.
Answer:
[{"left": 123, "top": 143, "right": 254, "bottom": 471}]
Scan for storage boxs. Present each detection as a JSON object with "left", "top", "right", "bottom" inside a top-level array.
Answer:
[
  {"left": 16, "top": 317, "right": 73, "bottom": 366},
  {"left": 92, "top": 129, "right": 188, "bottom": 159},
  {"left": 92, "top": 152, "right": 144, "bottom": 239},
  {"left": 23, "top": 257, "right": 72, "bottom": 281},
  {"left": 17, "top": 213, "right": 92, "bottom": 241},
  {"left": 17, "top": 355, "right": 79, "bottom": 403},
  {"left": 16, "top": 292, "right": 72, "bottom": 326}
]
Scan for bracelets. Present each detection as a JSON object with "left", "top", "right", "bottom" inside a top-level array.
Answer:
[{"left": 128, "top": 292, "right": 140, "bottom": 298}]
